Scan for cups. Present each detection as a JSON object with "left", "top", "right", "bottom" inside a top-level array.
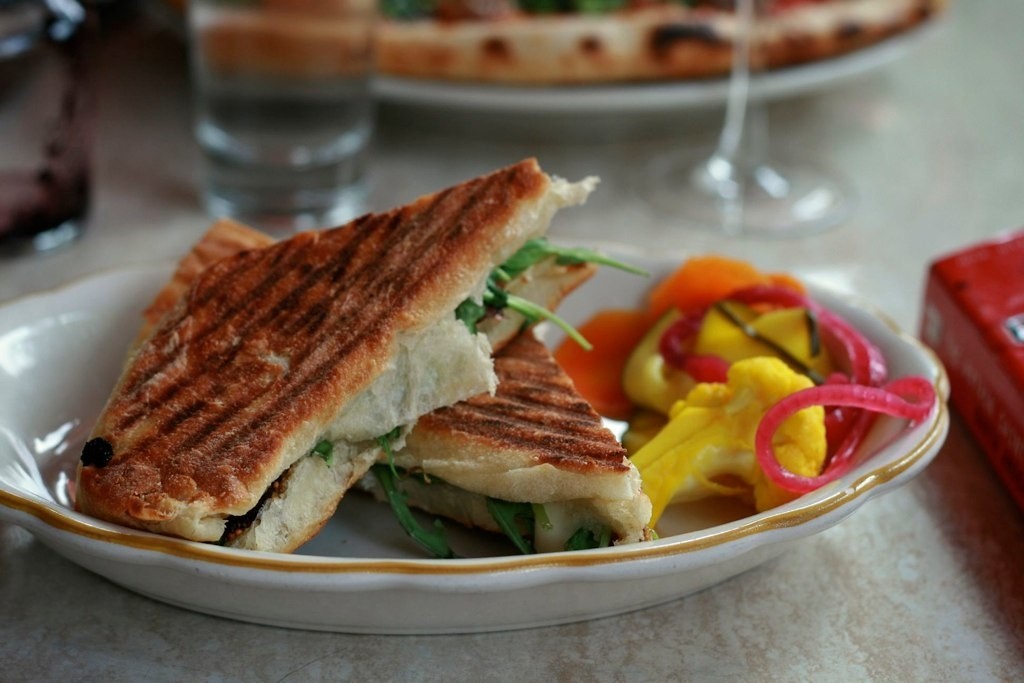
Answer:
[
  {"left": 0, "top": 0, "right": 96, "bottom": 257},
  {"left": 182, "top": 0, "right": 385, "bottom": 236}
]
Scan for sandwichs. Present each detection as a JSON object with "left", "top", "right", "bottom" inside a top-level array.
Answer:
[{"left": 74, "top": 156, "right": 653, "bottom": 568}]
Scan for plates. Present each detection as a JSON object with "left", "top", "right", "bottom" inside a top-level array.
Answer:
[
  {"left": 0, "top": 245, "right": 946, "bottom": 634},
  {"left": 206, "top": 1, "right": 961, "bottom": 134}
]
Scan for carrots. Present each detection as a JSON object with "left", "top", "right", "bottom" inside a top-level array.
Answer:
[{"left": 553, "top": 256, "right": 805, "bottom": 417}]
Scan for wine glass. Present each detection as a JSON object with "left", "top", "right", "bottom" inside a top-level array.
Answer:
[{"left": 637, "top": 4, "right": 858, "bottom": 239}]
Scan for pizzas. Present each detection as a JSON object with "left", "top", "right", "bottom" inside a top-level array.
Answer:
[{"left": 203, "top": 0, "right": 950, "bottom": 86}]
